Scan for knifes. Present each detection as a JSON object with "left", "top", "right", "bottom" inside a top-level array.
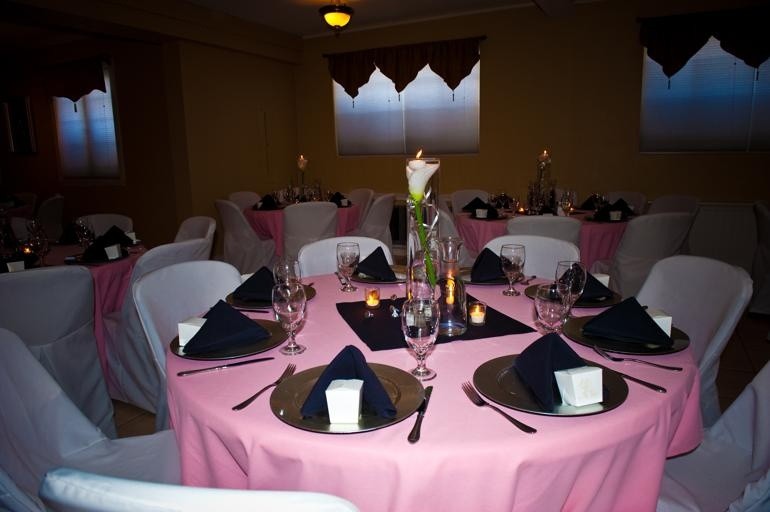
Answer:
[
  {"left": 612, "top": 369, "right": 666, "bottom": 392},
  {"left": 408, "top": 386, "right": 433, "bottom": 442},
  {"left": 177, "top": 357, "right": 274, "bottom": 376}
]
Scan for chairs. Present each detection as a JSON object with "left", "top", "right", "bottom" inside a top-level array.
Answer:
[
  {"left": 0, "top": 328, "right": 181, "bottom": 512},
  {"left": 656, "top": 356, "right": 770, "bottom": 512},
  {"left": 39, "top": 465, "right": 355, "bottom": 512}
]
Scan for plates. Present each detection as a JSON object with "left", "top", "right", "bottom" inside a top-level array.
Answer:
[
  {"left": 226, "top": 284, "right": 315, "bottom": 308},
  {"left": 170, "top": 319, "right": 288, "bottom": 360},
  {"left": 474, "top": 354, "right": 628, "bottom": 417},
  {"left": 562, "top": 315, "right": 691, "bottom": 355},
  {"left": 456, "top": 267, "right": 524, "bottom": 285},
  {"left": 270, "top": 362, "right": 426, "bottom": 434},
  {"left": 525, "top": 282, "right": 622, "bottom": 307},
  {"left": 346, "top": 270, "right": 407, "bottom": 283}
]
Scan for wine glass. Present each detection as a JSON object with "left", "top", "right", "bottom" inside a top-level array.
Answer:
[
  {"left": 402, "top": 299, "right": 441, "bottom": 381},
  {"left": 336, "top": 242, "right": 360, "bottom": 292},
  {"left": 561, "top": 196, "right": 573, "bottom": 217},
  {"left": 272, "top": 183, "right": 295, "bottom": 206},
  {"left": 271, "top": 283, "right": 307, "bottom": 355},
  {"left": 488, "top": 193, "right": 519, "bottom": 220},
  {"left": 500, "top": 244, "right": 525, "bottom": 296},
  {"left": 74, "top": 215, "right": 93, "bottom": 248},
  {"left": 535, "top": 284, "right": 573, "bottom": 332},
  {"left": 556, "top": 261, "right": 587, "bottom": 319},
  {"left": 273, "top": 260, "right": 301, "bottom": 312},
  {"left": 591, "top": 191, "right": 609, "bottom": 213},
  {"left": 303, "top": 182, "right": 335, "bottom": 203},
  {"left": 25, "top": 217, "right": 48, "bottom": 267}
]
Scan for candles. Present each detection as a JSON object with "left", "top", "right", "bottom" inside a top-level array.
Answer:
[{"left": 406, "top": 149, "right": 439, "bottom": 289}]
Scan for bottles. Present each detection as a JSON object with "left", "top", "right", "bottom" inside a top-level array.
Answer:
[{"left": 433, "top": 237, "right": 468, "bottom": 338}]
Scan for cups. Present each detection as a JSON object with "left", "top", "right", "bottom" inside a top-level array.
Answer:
[
  {"left": 526, "top": 183, "right": 541, "bottom": 215},
  {"left": 469, "top": 301, "right": 488, "bottom": 326},
  {"left": 363, "top": 286, "right": 380, "bottom": 309}
]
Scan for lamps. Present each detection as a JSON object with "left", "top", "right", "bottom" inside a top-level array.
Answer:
[{"left": 318, "top": 0, "right": 355, "bottom": 39}]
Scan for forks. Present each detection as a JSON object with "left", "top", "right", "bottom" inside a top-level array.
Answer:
[
  {"left": 593, "top": 343, "right": 683, "bottom": 371},
  {"left": 461, "top": 381, "right": 537, "bottom": 433},
  {"left": 232, "top": 363, "right": 296, "bottom": 412}
]
